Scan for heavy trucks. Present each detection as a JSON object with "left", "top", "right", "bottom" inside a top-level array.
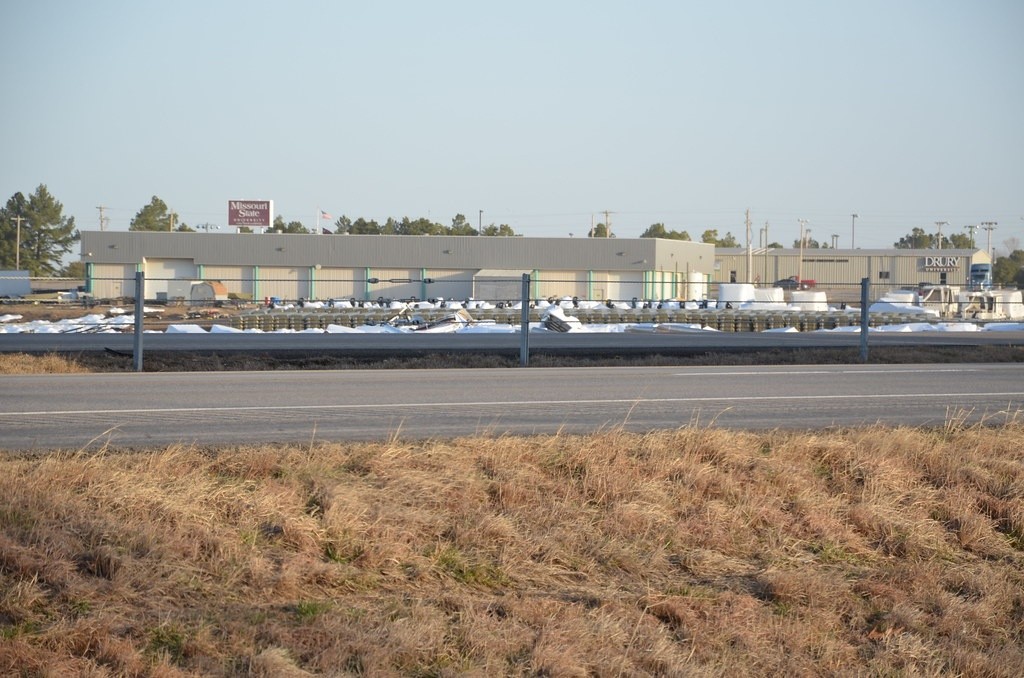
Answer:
[{"left": 970, "top": 263, "right": 994, "bottom": 292}]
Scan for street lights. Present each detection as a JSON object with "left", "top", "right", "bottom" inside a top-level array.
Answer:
[
  {"left": 479, "top": 210, "right": 484, "bottom": 235},
  {"left": 852, "top": 214, "right": 859, "bottom": 248},
  {"left": 798, "top": 218, "right": 810, "bottom": 287}
]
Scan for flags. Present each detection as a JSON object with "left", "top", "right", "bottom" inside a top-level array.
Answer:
[{"left": 321, "top": 211, "right": 331, "bottom": 219}]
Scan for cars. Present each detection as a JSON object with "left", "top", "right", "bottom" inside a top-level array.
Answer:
[{"left": 773, "top": 280, "right": 809, "bottom": 291}]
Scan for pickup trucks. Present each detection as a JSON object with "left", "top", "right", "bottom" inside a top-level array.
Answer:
[{"left": 785, "top": 276, "right": 815, "bottom": 288}]
